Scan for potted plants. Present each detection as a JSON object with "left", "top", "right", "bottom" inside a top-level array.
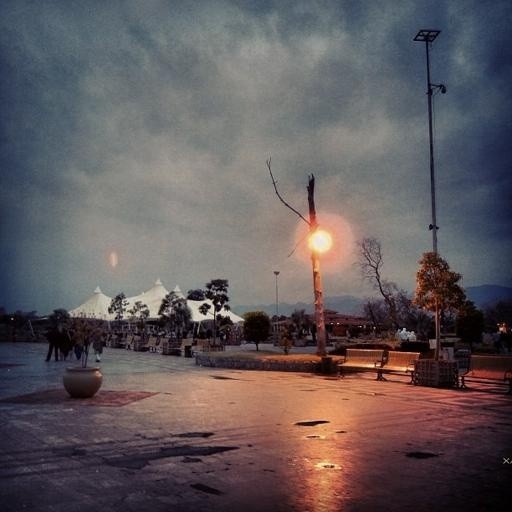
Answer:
[{"left": 62, "top": 310, "right": 104, "bottom": 398}]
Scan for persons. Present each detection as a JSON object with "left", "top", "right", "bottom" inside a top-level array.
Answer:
[
  {"left": 43, "top": 323, "right": 107, "bottom": 362},
  {"left": 392, "top": 326, "right": 419, "bottom": 342}
]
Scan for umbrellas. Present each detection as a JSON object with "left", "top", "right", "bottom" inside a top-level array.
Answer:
[
  {"left": 184, "top": 289, "right": 246, "bottom": 325},
  {"left": 119, "top": 279, "right": 170, "bottom": 319},
  {"left": 68, "top": 286, "right": 134, "bottom": 322},
  {"left": 160, "top": 282, "right": 187, "bottom": 318}
]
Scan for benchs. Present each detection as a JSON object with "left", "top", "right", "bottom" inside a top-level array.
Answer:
[
  {"left": 336, "top": 348, "right": 512, "bottom": 395},
  {"left": 117, "top": 335, "right": 221, "bottom": 358}
]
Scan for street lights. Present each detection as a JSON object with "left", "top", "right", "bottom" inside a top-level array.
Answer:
[
  {"left": 413, "top": 24, "right": 451, "bottom": 360},
  {"left": 273, "top": 271, "right": 280, "bottom": 317}
]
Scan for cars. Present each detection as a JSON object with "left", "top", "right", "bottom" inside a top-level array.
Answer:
[{"left": 304, "top": 333, "right": 316, "bottom": 341}]
